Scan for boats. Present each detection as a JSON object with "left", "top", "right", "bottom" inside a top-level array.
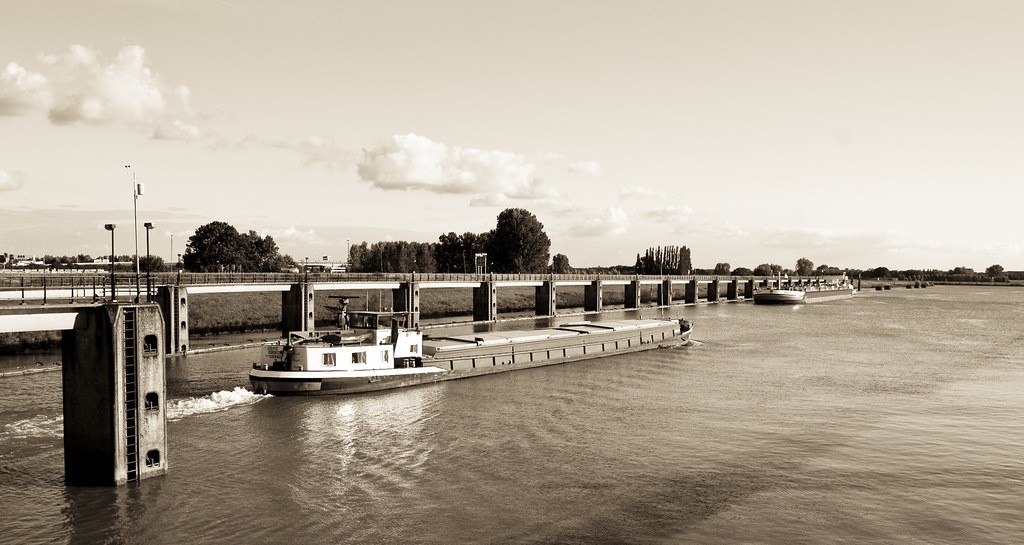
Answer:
[
  {"left": 753, "top": 288, "right": 806, "bottom": 304},
  {"left": 249, "top": 293, "right": 694, "bottom": 397}
]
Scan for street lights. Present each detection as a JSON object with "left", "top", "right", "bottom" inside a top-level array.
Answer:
[
  {"left": 144, "top": 222, "right": 156, "bottom": 302},
  {"left": 346, "top": 240, "right": 350, "bottom": 258},
  {"left": 171, "top": 233, "right": 174, "bottom": 273},
  {"left": 105, "top": 223, "right": 116, "bottom": 301},
  {"left": 305, "top": 257, "right": 309, "bottom": 283}
]
